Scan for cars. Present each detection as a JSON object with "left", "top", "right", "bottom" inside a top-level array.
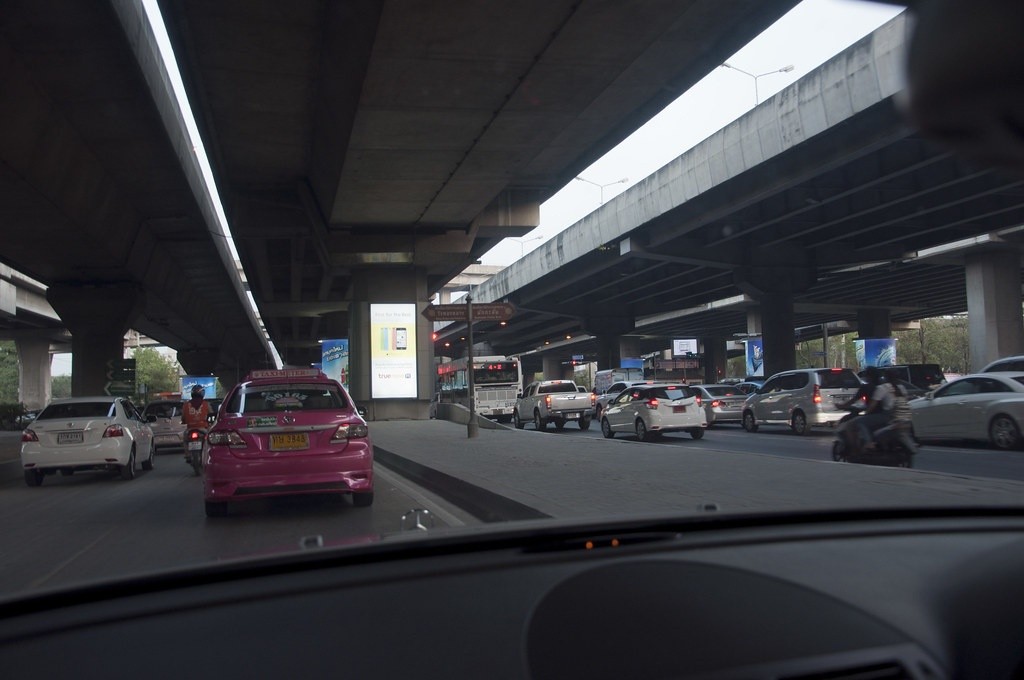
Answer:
[
  {"left": 19, "top": 394, "right": 157, "bottom": 487},
  {"left": 600, "top": 383, "right": 707, "bottom": 442},
  {"left": 733, "top": 380, "right": 767, "bottom": 396},
  {"left": 903, "top": 370, "right": 1024, "bottom": 450},
  {"left": 141, "top": 399, "right": 188, "bottom": 446},
  {"left": 690, "top": 384, "right": 751, "bottom": 431},
  {"left": 977, "top": 354, "right": 1024, "bottom": 373},
  {"left": 596, "top": 381, "right": 646, "bottom": 423}
]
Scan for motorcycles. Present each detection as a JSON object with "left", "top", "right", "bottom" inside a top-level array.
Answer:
[
  {"left": 830, "top": 402, "right": 921, "bottom": 468},
  {"left": 180, "top": 422, "right": 209, "bottom": 477}
]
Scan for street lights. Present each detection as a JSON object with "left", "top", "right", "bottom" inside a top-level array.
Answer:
[
  {"left": 575, "top": 176, "right": 630, "bottom": 205},
  {"left": 719, "top": 60, "right": 796, "bottom": 108},
  {"left": 506, "top": 236, "right": 544, "bottom": 258}
]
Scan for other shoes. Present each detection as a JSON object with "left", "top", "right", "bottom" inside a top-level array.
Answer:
[
  {"left": 186, "top": 456, "right": 191, "bottom": 463},
  {"left": 863, "top": 442, "right": 876, "bottom": 449}
]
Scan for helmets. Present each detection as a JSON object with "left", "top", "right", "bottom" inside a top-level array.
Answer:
[{"left": 191, "top": 385, "right": 204, "bottom": 398}]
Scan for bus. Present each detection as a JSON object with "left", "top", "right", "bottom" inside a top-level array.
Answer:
[{"left": 437, "top": 353, "right": 523, "bottom": 422}]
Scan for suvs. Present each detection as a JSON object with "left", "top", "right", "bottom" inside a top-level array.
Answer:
[
  {"left": 200, "top": 367, "right": 375, "bottom": 518},
  {"left": 857, "top": 362, "right": 948, "bottom": 391},
  {"left": 739, "top": 365, "right": 867, "bottom": 437}
]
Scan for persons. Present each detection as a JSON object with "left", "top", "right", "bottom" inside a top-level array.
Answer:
[
  {"left": 835, "top": 366, "right": 909, "bottom": 458},
  {"left": 181, "top": 384, "right": 214, "bottom": 459}
]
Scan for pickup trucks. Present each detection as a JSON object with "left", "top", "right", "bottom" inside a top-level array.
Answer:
[{"left": 513, "top": 379, "right": 596, "bottom": 432}]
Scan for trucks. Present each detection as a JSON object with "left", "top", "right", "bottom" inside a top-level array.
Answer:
[{"left": 592, "top": 367, "right": 644, "bottom": 395}]
